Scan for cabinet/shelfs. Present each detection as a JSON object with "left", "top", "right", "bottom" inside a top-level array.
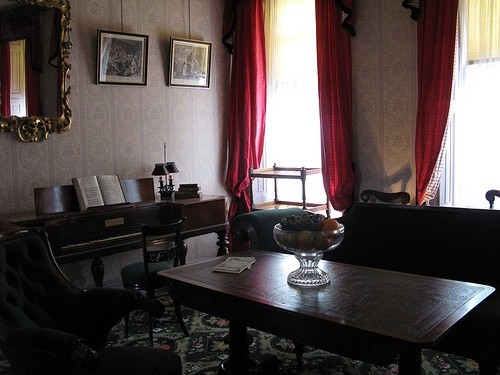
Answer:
[{"left": 250, "top": 163, "right": 331, "bottom": 218}]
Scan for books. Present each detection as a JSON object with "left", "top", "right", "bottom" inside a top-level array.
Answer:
[
  {"left": 213, "top": 256, "right": 256, "bottom": 273},
  {"left": 71, "top": 174, "right": 127, "bottom": 212}
]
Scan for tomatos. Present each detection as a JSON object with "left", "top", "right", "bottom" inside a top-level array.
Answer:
[{"left": 278, "top": 229, "right": 331, "bottom": 250}]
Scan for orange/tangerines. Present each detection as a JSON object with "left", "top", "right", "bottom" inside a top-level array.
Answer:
[{"left": 322, "top": 219, "right": 339, "bottom": 231}]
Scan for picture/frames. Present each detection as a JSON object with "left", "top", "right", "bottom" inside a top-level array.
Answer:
[
  {"left": 168, "top": 37, "right": 213, "bottom": 88},
  {"left": 96, "top": 28, "right": 149, "bottom": 86}
]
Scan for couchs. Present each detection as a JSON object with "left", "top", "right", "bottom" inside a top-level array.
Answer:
[{"left": 230, "top": 201, "right": 500, "bottom": 357}]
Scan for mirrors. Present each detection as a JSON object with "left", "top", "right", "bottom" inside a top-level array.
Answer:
[{"left": 0, "top": 0, "right": 73, "bottom": 142}]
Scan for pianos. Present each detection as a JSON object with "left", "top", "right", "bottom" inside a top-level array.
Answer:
[{"left": 0, "top": 194, "right": 229, "bottom": 288}]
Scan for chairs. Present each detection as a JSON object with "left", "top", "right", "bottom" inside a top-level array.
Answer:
[{"left": 0, "top": 226, "right": 182, "bottom": 375}]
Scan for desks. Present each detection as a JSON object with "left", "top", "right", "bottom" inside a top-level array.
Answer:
[
  {"left": 156, "top": 250, "right": 495, "bottom": 375},
  {"left": 0, "top": 194, "right": 230, "bottom": 287}
]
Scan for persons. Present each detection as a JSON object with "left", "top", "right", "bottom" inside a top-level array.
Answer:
[
  {"left": 102, "top": 48, "right": 138, "bottom": 76},
  {"left": 174, "top": 47, "right": 205, "bottom": 78}
]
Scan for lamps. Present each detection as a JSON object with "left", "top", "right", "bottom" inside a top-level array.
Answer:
[{"left": 151, "top": 160, "right": 179, "bottom": 200}]
